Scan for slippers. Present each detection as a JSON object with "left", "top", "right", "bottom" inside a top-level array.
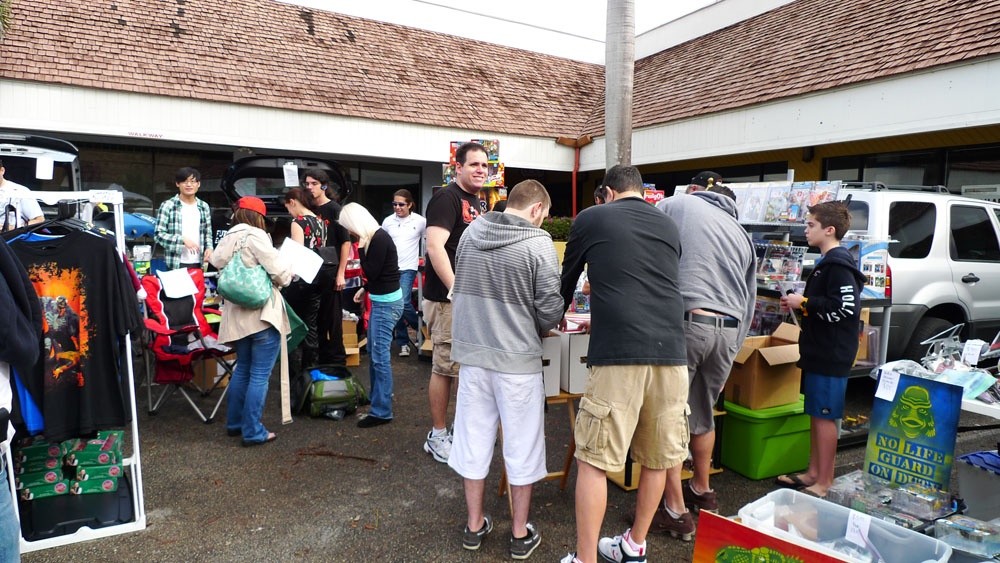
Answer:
[
  {"left": 776, "top": 473, "right": 806, "bottom": 489},
  {"left": 799, "top": 487, "right": 821, "bottom": 499}
]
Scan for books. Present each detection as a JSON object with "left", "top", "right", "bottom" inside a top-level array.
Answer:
[{"left": 746, "top": 239, "right": 809, "bottom": 337}]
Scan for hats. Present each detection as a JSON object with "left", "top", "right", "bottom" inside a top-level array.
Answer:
[
  {"left": 238, "top": 196, "right": 275, "bottom": 226},
  {"left": 692, "top": 171, "right": 723, "bottom": 191}
]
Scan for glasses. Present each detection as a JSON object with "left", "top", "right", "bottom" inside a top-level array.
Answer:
[{"left": 392, "top": 201, "right": 409, "bottom": 207}]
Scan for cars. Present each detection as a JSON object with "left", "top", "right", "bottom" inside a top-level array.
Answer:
[
  {"left": 0, "top": 134, "right": 82, "bottom": 222},
  {"left": 211, "top": 155, "right": 424, "bottom": 341}
]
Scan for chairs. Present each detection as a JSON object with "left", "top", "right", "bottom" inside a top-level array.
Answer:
[{"left": 141, "top": 267, "right": 234, "bottom": 423}]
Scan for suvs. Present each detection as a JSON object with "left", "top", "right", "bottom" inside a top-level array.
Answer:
[{"left": 739, "top": 179, "right": 1000, "bottom": 381}]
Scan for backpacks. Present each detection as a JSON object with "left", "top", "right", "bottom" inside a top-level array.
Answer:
[{"left": 307, "top": 216, "right": 340, "bottom": 277}]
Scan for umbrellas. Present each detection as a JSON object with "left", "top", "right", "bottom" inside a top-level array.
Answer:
[{"left": 123, "top": 211, "right": 156, "bottom": 246}]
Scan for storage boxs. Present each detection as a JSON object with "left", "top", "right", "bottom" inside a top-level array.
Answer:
[
  {"left": 342, "top": 320, "right": 360, "bottom": 366},
  {"left": 187, "top": 353, "right": 237, "bottom": 389},
  {"left": 542, "top": 329, "right": 590, "bottom": 397},
  {"left": 721, "top": 322, "right": 811, "bottom": 480},
  {"left": 738, "top": 488, "right": 952, "bottom": 563}
]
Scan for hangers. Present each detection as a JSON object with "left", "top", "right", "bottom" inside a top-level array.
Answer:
[{"left": 0, "top": 196, "right": 113, "bottom": 249}]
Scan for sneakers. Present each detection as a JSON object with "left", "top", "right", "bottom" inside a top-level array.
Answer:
[
  {"left": 681, "top": 480, "right": 719, "bottom": 515},
  {"left": 560, "top": 551, "right": 582, "bottom": 563},
  {"left": 630, "top": 504, "right": 696, "bottom": 541},
  {"left": 510, "top": 520, "right": 541, "bottom": 559},
  {"left": 463, "top": 514, "right": 494, "bottom": 550},
  {"left": 423, "top": 430, "right": 452, "bottom": 464},
  {"left": 448, "top": 428, "right": 454, "bottom": 443},
  {"left": 598, "top": 526, "right": 647, "bottom": 563}
]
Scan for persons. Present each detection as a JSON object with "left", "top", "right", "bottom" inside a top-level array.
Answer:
[
  {"left": 447, "top": 179, "right": 563, "bottom": 559},
  {"left": 382, "top": 190, "right": 421, "bottom": 356},
  {"left": 154, "top": 167, "right": 214, "bottom": 271},
  {"left": 555, "top": 165, "right": 693, "bottom": 563},
  {"left": 492, "top": 200, "right": 506, "bottom": 212},
  {"left": 303, "top": 168, "right": 351, "bottom": 364},
  {"left": 0, "top": 159, "right": 45, "bottom": 231},
  {"left": 210, "top": 196, "right": 291, "bottom": 446},
  {"left": 280, "top": 186, "right": 327, "bottom": 408},
  {"left": 686, "top": 171, "right": 720, "bottom": 194},
  {"left": 576, "top": 273, "right": 590, "bottom": 295},
  {"left": 424, "top": 143, "right": 488, "bottom": 463},
  {"left": 627, "top": 185, "right": 756, "bottom": 542},
  {"left": 593, "top": 186, "right": 605, "bottom": 204},
  {"left": 778, "top": 202, "right": 867, "bottom": 496},
  {"left": 338, "top": 202, "right": 404, "bottom": 427}
]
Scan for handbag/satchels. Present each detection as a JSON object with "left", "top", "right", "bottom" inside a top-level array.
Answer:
[{"left": 217, "top": 234, "right": 272, "bottom": 309}]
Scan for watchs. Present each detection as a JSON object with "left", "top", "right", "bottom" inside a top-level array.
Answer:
[{"left": 800, "top": 298, "right": 808, "bottom": 316}]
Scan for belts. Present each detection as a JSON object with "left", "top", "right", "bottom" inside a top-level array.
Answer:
[{"left": 684, "top": 312, "right": 738, "bottom": 327}]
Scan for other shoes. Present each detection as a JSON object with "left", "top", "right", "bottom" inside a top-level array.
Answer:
[
  {"left": 243, "top": 432, "right": 276, "bottom": 445},
  {"left": 399, "top": 344, "right": 411, "bottom": 356},
  {"left": 357, "top": 415, "right": 391, "bottom": 428},
  {"left": 227, "top": 429, "right": 242, "bottom": 437}
]
